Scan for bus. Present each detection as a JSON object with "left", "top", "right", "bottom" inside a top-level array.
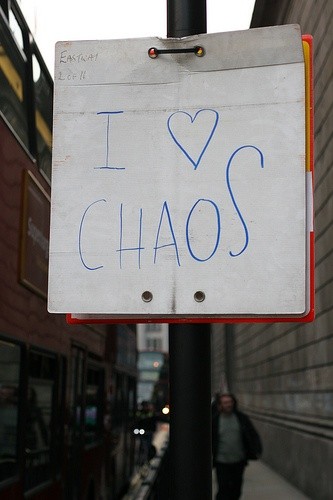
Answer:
[{"left": 1, "top": 0, "right": 136, "bottom": 499}]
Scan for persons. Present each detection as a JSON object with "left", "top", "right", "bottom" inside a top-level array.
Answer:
[
  {"left": 131, "top": 401, "right": 152, "bottom": 469},
  {"left": 126, "top": 400, "right": 156, "bottom": 437},
  {"left": 207, "top": 395, "right": 263, "bottom": 500}
]
